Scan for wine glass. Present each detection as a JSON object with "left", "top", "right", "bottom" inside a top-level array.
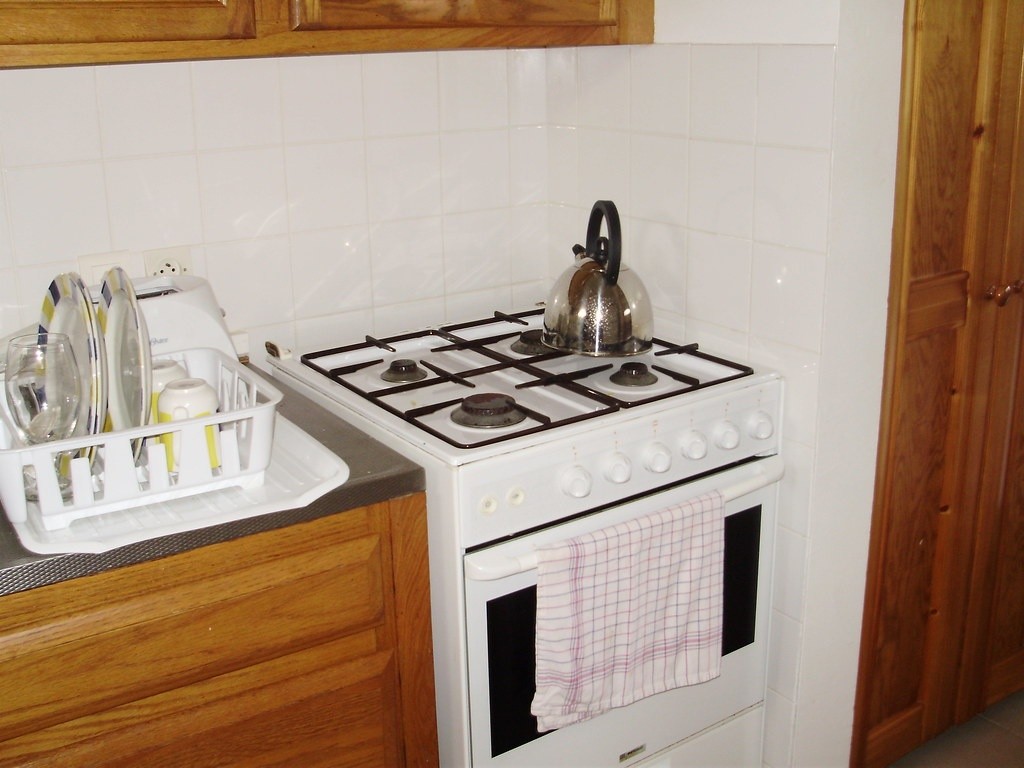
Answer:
[{"left": 7, "top": 331, "right": 79, "bottom": 494}]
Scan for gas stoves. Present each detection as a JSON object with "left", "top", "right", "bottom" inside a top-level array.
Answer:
[{"left": 302, "top": 301, "right": 786, "bottom": 548}]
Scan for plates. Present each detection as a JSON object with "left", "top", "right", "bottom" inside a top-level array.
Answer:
[
  {"left": 97, "top": 267, "right": 153, "bottom": 468},
  {"left": 39, "top": 269, "right": 106, "bottom": 482}
]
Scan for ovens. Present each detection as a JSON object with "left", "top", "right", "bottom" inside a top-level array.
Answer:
[{"left": 452, "top": 455, "right": 822, "bottom": 768}]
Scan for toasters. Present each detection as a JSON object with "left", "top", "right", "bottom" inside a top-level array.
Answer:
[{"left": 86, "top": 274, "right": 250, "bottom": 409}]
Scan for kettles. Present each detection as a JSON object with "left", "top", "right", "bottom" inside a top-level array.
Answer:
[{"left": 543, "top": 201, "right": 656, "bottom": 357}]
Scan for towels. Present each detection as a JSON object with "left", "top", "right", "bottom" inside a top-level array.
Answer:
[{"left": 529, "top": 488, "right": 727, "bottom": 735}]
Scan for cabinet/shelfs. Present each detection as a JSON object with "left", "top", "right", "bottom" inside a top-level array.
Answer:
[
  {"left": 847, "top": 0, "right": 1024, "bottom": 768},
  {"left": 0, "top": 494, "right": 442, "bottom": 768},
  {"left": 0, "top": 0, "right": 688, "bottom": 76}
]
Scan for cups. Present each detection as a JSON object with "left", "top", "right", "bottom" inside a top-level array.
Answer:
[
  {"left": 138, "top": 358, "right": 184, "bottom": 436},
  {"left": 156, "top": 378, "right": 222, "bottom": 476}
]
